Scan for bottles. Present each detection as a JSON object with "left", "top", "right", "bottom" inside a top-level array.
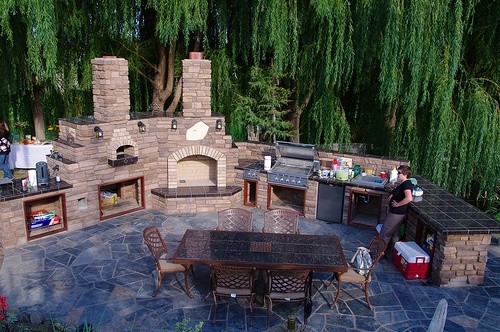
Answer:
[
  {"left": 390, "top": 166, "right": 398, "bottom": 182},
  {"left": 318, "top": 159, "right": 359, "bottom": 181},
  {"left": 56, "top": 173, "right": 60, "bottom": 182},
  {"left": 22, "top": 176, "right": 31, "bottom": 192}
]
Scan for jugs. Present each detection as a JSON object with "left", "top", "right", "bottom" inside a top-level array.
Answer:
[{"left": 36, "top": 161, "right": 49, "bottom": 186}]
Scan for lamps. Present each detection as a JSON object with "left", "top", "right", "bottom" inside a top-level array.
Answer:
[
  {"left": 138, "top": 122, "right": 146, "bottom": 134},
  {"left": 172, "top": 119, "right": 177, "bottom": 131},
  {"left": 216, "top": 119, "right": 222, "bottom": 131},
  {"left": 94, "top": 126, "right": 103, "bottom": 140}
]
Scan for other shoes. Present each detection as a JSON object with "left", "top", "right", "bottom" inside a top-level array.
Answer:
[{"left": 1, "top": 176, "right": 15, "bottom": 181}]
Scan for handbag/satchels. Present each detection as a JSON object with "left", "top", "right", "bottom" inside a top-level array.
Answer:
[{"left": 350, "top": 247, "right": 372, "bottom": 275}]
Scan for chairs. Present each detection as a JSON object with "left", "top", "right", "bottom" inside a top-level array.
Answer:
[
  {"left": 326, "top": 236, "right": 387, "bottom": 312},
  {"left": 143, "top": 227, "right": 200, "bottom": 299},
  {"left": 204, "top": 208, "right": 259, "bottom": 327},
  {"left": 260, "top": 209, "right": 313, "bottom": 332}
]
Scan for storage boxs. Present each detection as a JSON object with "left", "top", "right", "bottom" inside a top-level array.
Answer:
[
  {"left": 100, "top": 195, "right": 118, "bottom": 206},
  {"left": 393, "top": 241, "right": 430, "bottom": 279}
]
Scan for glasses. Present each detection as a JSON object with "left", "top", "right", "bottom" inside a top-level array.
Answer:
[{"left": 398, "top": 171, "right": 402, "bottom": 175}]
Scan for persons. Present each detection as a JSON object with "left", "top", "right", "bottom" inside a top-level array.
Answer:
[
  {"left": 0, "top": 122, "right": 16, "bottom": 183},
  {"left": 377, "top": 164, "right": 412, "bottom": 259}
]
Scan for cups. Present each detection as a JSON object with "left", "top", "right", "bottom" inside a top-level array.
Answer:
[
  {"left": 264, "top": 156, "right": 271, "bottom": 170},
  {"left": 22, "top": 180, "right": 26, "bottom": 186},
  {"left": 28, "top": 169, "right": 36, "bottom": 186},
  {"left": 380, "top": 172, "right": 385, "bottom": 178}
]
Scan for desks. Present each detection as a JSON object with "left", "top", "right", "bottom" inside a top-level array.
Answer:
[
  {"left": 173, "top": 229, "right": 348, "bottom": 310},
  {"left": 9, "top": 142, "right": 53, "bottom": 169}
]
